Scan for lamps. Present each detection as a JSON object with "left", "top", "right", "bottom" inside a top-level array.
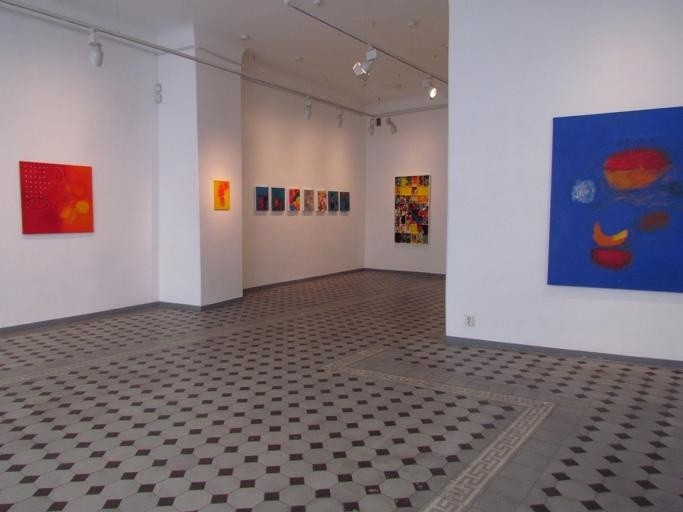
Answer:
[
  {"left": 302, "top": 94, "right": 312, "bottom": 121},
  {"left": 85, "top": 24, "right": 106, "bottom": 68},
  {"left": 368, "top": 116, "right": 376, "bottom": 136},
  {"left": 421, "top": 72, "right": 438, "bottom": 99},
  {"left": 335, "top": 104, "right": 343, "bottom": 128},
  {"left": 352, "top": 42, "right": 379, "bottom": 86},
  {"left": 385, "top": 117, "right": 397, "bottom": 134}
]
